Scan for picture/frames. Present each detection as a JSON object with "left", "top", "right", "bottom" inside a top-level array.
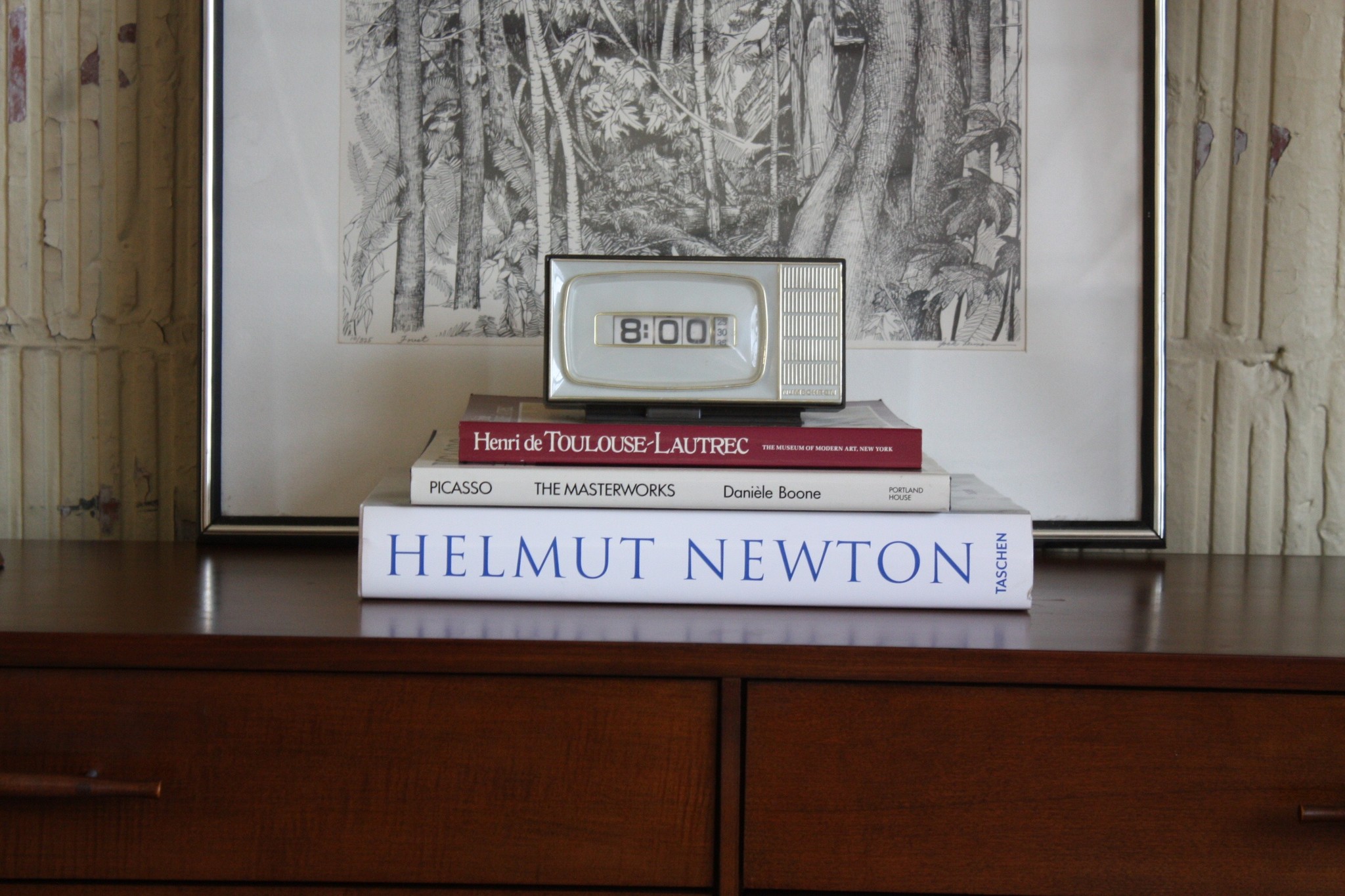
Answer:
[{"left": 195, "top": 0, "right": 1178, "bottom": 562}]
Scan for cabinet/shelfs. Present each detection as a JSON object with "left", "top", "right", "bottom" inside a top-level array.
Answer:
[{"left": 0, "top": 530, "right": 1345, "bottom": 896}]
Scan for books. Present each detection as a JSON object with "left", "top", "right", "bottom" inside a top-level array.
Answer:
[{"left": 357, "top": 395, "right": 1034, "bottom": 609}]
